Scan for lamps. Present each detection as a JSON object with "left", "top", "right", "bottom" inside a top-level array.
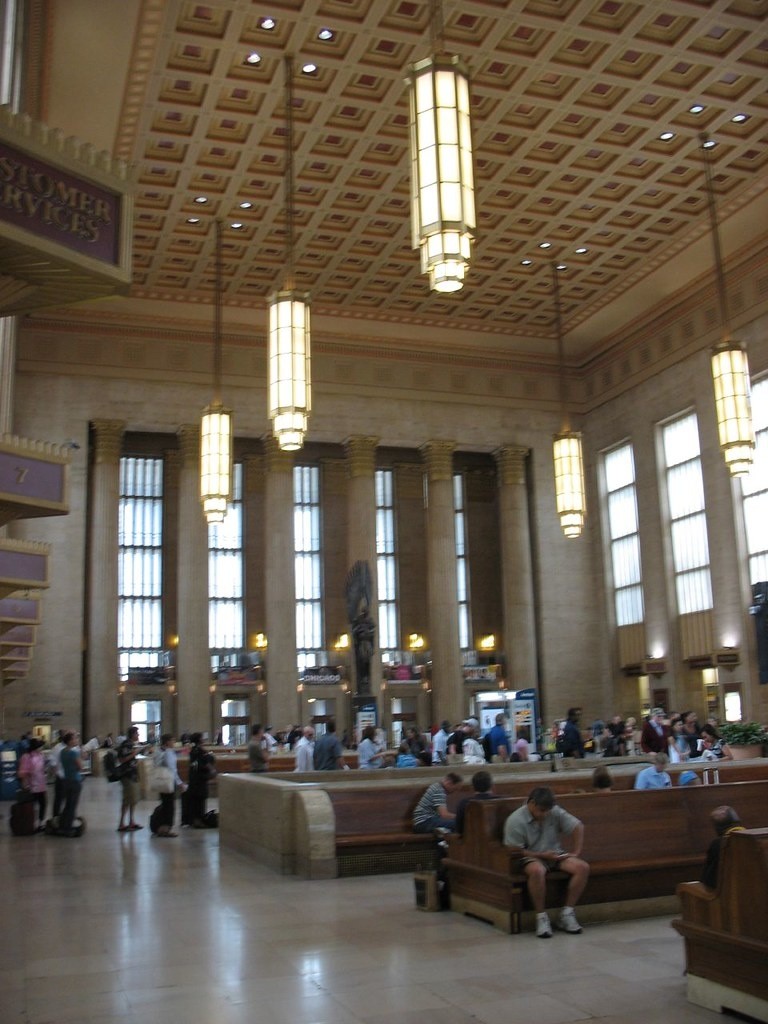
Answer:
[
  {"left": 421, "top": 677, "right": 433, "bottom": 696},
  {"left": 697, "top": 130, "right": 739, "bottom": 480},
  {"left": 256, "top": 681, "right": 271, "bottom": 697},
  {"left": 497, "top": 676, "right": 513, "bottom": 691},
  {"left": 641, "top": 654, "right": 668, "bottom": 677},
  {"left": 339, "top": 678, "right": 358, "bottom": 697},
  {"left": 408, "top": 0, "right": 473, "bottom": 297},
  {"left": 115, "top": 682, "right": 126, "bottom": 697},
  {"left": 712, "top": 644, "right": 744, "bottom": 666},
  {"left": 551, "top": 263, "right": 587, "bottom": 540},
  {"left": 165, "top": 680, "right": 183, "bottom": 698},
  {"left": 296, "top": 681, "right": 304, "bottom": 694},
  {"left": 202, "top": 214, "right": 233, "bottom": 528},
  {"left": 208, "top": 680, "right": 217, "bottom": 696},
  {"left": 266, "top": 52, "right": 321, "bottom": 453}
]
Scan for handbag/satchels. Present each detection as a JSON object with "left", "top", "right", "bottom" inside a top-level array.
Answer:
[
  {"left": 414, "top": 862, "right": 442, "bottom": 912},
  {"left": 202, "top": 809, "right": 220, "bottom": 828},
  {"left": 150, "top": 748, "right": 174, "bottom": 793},
  {"left": 45, "top": 815, "right": 87, "bottom": 837},
  {"left": 203, "top": 754, "right": 218, "bottom": 778}
]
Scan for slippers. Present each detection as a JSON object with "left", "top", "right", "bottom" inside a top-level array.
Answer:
[{"left": 117, "top": 825, "right": 143, "bottom": 831}]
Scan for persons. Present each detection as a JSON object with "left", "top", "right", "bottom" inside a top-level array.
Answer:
[
  {"left": 0, "top": 706, "right": 733, "bottom": 871},
  {"left": 694, "top": 804, "right": 747, "bottom": 890},
  {"left": 351, "top": 604, "right": 377, "bottom": 686},
  {"left": 503, "top": 787, "right": 590, "bottom": 937}
]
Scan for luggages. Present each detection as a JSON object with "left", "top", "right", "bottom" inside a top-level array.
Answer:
[
  {"left": 10, "top": 803, "right": 38, "bottom": 835},
  {"left": 180, "top": 789, "right": 199, "bottom": 828}
]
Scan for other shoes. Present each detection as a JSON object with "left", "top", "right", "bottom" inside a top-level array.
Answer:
[
  {"left": 437, "top": 840, "right": 449, "bottom": 849},
  {"left": 434, "top": 827, "right": 452, "bottom": 839}
]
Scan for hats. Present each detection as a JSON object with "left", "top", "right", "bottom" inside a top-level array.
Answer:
[
  {"left": 19, "top": 739, "right": 46, "bottom": 752},
  {"left": 652, "top": 708, "right": 667, "bottom": 715},
  {"left": 463, "top": 718, "right": 479, "bottom": 726},
  {"left": 677, "top": 770, "right": 699, "bottom": 785},
  {"left": 656, "top": 752, "right": 668, "bottom": 764}
]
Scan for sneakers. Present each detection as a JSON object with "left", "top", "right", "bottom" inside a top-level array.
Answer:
[
  {"left": 554, "top": 912, "right": 584, "bottom": 934},
  {"left": 536, "top": 917, "right": 553, "bottom": 937}
]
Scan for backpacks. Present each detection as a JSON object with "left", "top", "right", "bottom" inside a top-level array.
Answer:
[
  {"left": 14, "top": 789, "right": 28, "bottom": 802},
  {"left": 103, "top": 747, "right": 128, "bottom": 782},
  {"left": 150, "top": 802, "right": 171, "bottom": 837}
]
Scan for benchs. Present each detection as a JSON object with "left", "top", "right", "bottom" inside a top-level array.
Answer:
[{"left": 144, "top": 738, "right": 768, "bottom": 1024}]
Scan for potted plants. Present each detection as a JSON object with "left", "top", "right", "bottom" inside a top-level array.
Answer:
[{"left": 720, "top": 720, "right": 768, "bottom": 762}]
